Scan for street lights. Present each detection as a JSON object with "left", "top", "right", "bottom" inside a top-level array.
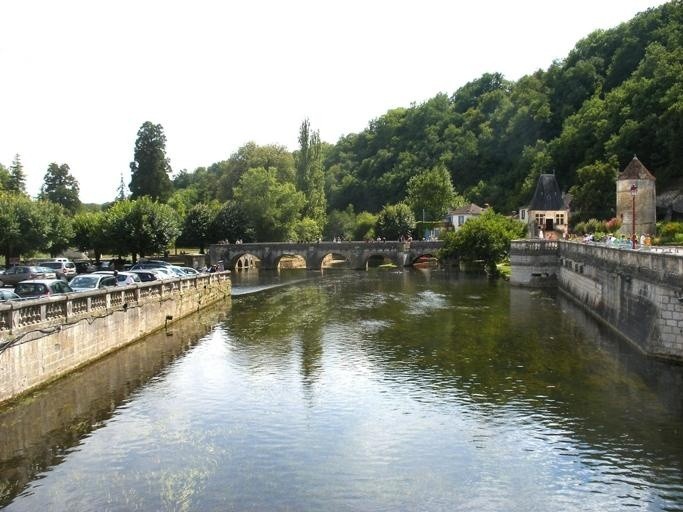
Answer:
[{"left": 631, "top": 184, "right": 638, "bottom": 249}]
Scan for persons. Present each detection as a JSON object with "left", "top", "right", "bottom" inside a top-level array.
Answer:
[
  {"left": 536, "top": 230, "right": 655, "bottom": 247},
  {"left": 200, "top": 264, "right": 220, "bottom": 273},
  {"left": 336, "top": 233, "right": 344, "bottom": 240},
  {"left": 377, "top": 235, "right": 381, "bottom": 240},
  {"left": 397, "top": 234, "right": 405, "bottom": 242},
  {"left": 407, "top": 234, "right": 412, "bottom": 241},
  {"left": 235, "top": 238, "right": 242, "bottom": 244},
  {"left": 218, "top": 238, "right": 229, "bottom": 244}
]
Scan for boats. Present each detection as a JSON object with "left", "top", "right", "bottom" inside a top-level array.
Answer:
[{"left": 379, "top": 263, "right": 398, "bottom": 269}]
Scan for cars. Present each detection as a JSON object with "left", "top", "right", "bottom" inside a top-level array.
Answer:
[{"left": 0, "top": 258, "right": 200, "bottom": 302}]
